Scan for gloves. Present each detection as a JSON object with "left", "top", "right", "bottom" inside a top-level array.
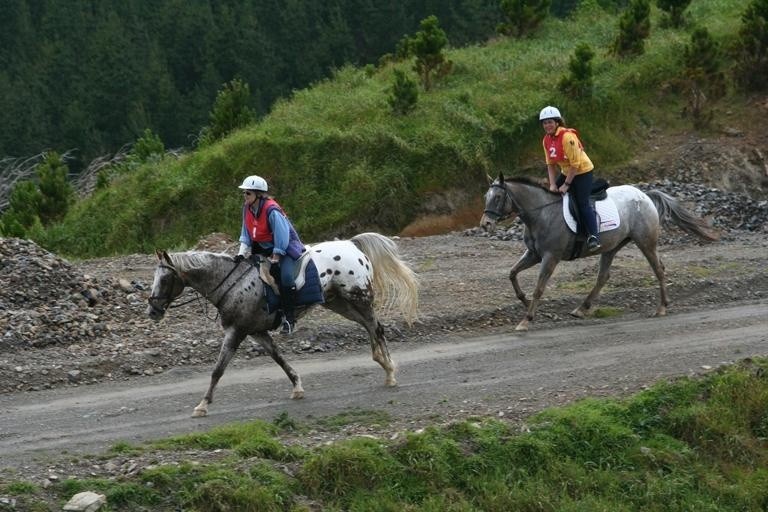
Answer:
[
  {"left": 232, "top": 254, "right": 246, "bottom": 264},
  {"left": 269, "top": 262, "right": 281, "bottom": 280}
]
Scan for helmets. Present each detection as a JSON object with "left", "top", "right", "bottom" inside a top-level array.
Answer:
[
  {"left": 238, "top": 175, "right": 268, "bottom": 193},
  {"left": 539, "top": 106, "right": 561, "bottom": 120}
]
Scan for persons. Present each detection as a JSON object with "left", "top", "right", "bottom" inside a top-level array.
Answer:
[
  {"left": 235, "top": 175, "right": 306, "bottom": 334},
  {"left": 540, "top": 105, "right": 598, "bottom": 249}
]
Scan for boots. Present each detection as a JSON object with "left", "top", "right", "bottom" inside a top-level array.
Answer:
[
  {"left": 279, "top": 284, "right": 297, "bottom": 335},
  {"left": 571, "top": 196, "right": 601, "bottom": 252}
]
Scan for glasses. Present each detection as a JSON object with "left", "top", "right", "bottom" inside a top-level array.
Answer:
[{"left": 243, "top": 191, "right": 253, "bottom": 196}]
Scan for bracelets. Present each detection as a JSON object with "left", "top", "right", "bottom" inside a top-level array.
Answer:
[{"left": 564, "top": 181, "right": 571, "bottom": 187}]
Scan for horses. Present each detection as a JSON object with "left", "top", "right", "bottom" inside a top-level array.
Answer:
[
  {"left": 479, "top": 170, "right": 721, "bottom": 331},
  {"left": 145, "top": 232, "right": 423, "bottom": 418}
]
